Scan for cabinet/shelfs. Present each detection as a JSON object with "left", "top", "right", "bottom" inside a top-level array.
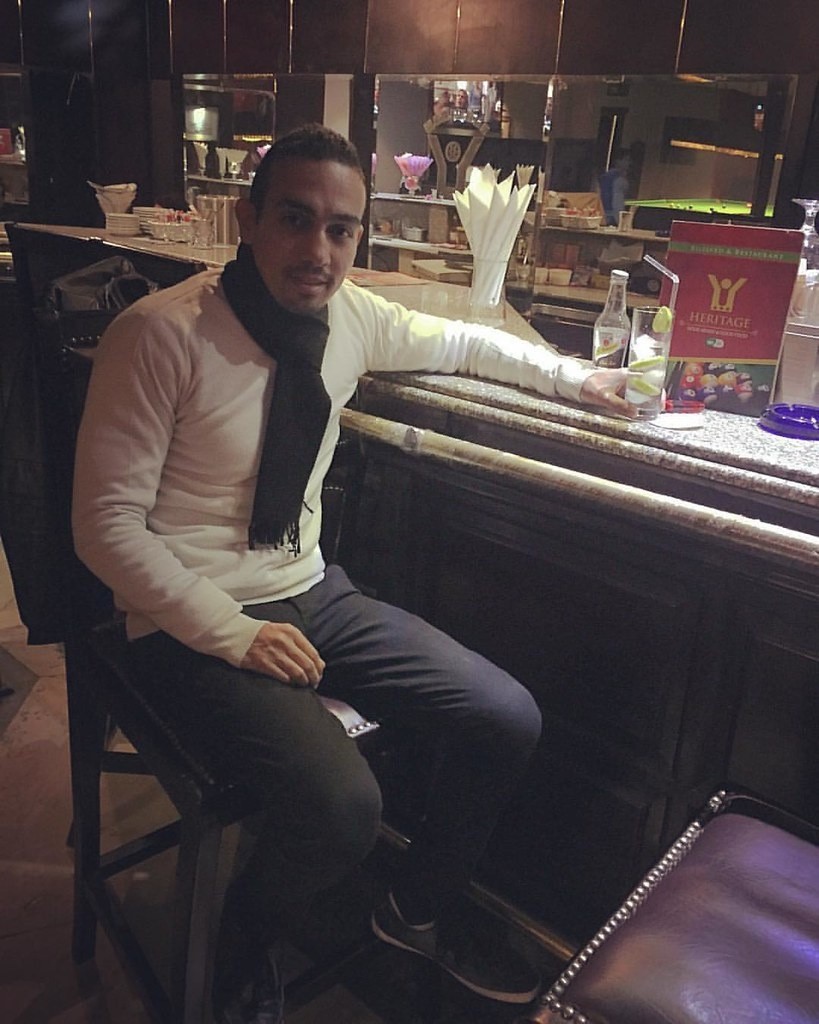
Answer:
[
  {"left": 0, "top": 0, "right": 33, "bottom": 285},
  {"left": 183, "top": 72, "right": 354, "bottom": 202},
  {"left": 367, "top": 73, "right": 797, "bottom": 362}
]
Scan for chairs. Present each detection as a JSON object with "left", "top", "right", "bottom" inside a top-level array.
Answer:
[
  {"left": 512, "top": 789, "right": 819, "bottom": 1024},
  {"left": 0, "top": 261, "right": 390, "bottom": 1024}
]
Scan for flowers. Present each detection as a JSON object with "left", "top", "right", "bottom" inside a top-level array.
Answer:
[{"left": 392, "top": 153, "right": 434, "bottom": 190}]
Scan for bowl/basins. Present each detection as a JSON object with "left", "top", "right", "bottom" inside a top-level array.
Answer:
[
  {"left": 147, "top": 219, "right": 192, "bottom": 241},
  {"left": 404, "top": 227, "right": 427, "bottom": 241},
  {"left": 549, "top": 269, "right": 572, "bottom": 286}
]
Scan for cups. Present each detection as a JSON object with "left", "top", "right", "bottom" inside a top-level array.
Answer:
[
  {"left": 624, "top": 306, "right": 675, "bottom": 420},
  {"left": 471, "top": 259, "right": 508, "bottom": 323},
  {"left": 188, "top": 220, "right": 214, "bottom": 250},
  {"left": 791, "top": 270, "right": 819, "bottom": 326},
  {"left": 516, "top": 263, "right": 529, "bottom": 281},
  {"left": 393, "top": 220, "right": 402, "bottom": 235}
]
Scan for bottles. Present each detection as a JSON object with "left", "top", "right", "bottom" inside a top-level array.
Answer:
[
  {"left": 501, "top": 105, "right": 511, "bottom": 139},
  {"left": 593, "top": 270, "right": 631, "bottom": 367},
  {"left": 754, "top": 104, "right": 765, "bottom": 131}
]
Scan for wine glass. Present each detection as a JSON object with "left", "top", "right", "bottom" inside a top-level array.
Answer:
[
  {"left": 405, "top": 175, "right": 421, "bottom": 200},
  {"left": 790, "top": 197, "right": 819, "bottom": 275},
  {"left": 225, "top": 161, "right": 244, "bottom": 182}
]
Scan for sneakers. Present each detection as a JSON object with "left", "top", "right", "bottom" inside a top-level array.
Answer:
[
  {"left": 223, "top": 956, "right": 282, "bottom": 1024},
  {"left": 372, "top": 883, "right": 541, "bottom": 1004}
]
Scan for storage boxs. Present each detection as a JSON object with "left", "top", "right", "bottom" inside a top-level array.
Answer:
[
  {"left": 0, "top": 129, "right": 14, "bottom": 154},
  {"left": 543, "top": 207, "right": 602, "bottom": 229}
]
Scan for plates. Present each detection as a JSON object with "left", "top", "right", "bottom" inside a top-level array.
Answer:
[{"left": 106, "top": 207, "right": 172, "bottom": 235}]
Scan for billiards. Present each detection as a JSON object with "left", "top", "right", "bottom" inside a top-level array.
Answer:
[{"left": 669, "top": 199, "right": 752, "bottom": 212}]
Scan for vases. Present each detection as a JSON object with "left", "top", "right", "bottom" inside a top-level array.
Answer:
[{"left": 401, "top": 191, "right": 425, "bottom": 199}]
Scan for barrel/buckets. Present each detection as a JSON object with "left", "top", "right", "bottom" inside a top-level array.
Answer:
[{"left": 197, "top": 194, "right": 240, "bottom": 244}]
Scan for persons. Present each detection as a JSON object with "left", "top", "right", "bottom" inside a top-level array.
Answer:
[
  {"left": 434, "top": 89, "right": 474, "bottom": 118},
  {"left": 70, "top": 122, "right": 666, "bottom": 1024},
  {"left": 598, "top": 147, "right": 633, "bottom": 228}
]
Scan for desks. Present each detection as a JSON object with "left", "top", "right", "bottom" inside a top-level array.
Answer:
[{"left": 320, "top": 368, "right": 819, "bottom": 960}]
